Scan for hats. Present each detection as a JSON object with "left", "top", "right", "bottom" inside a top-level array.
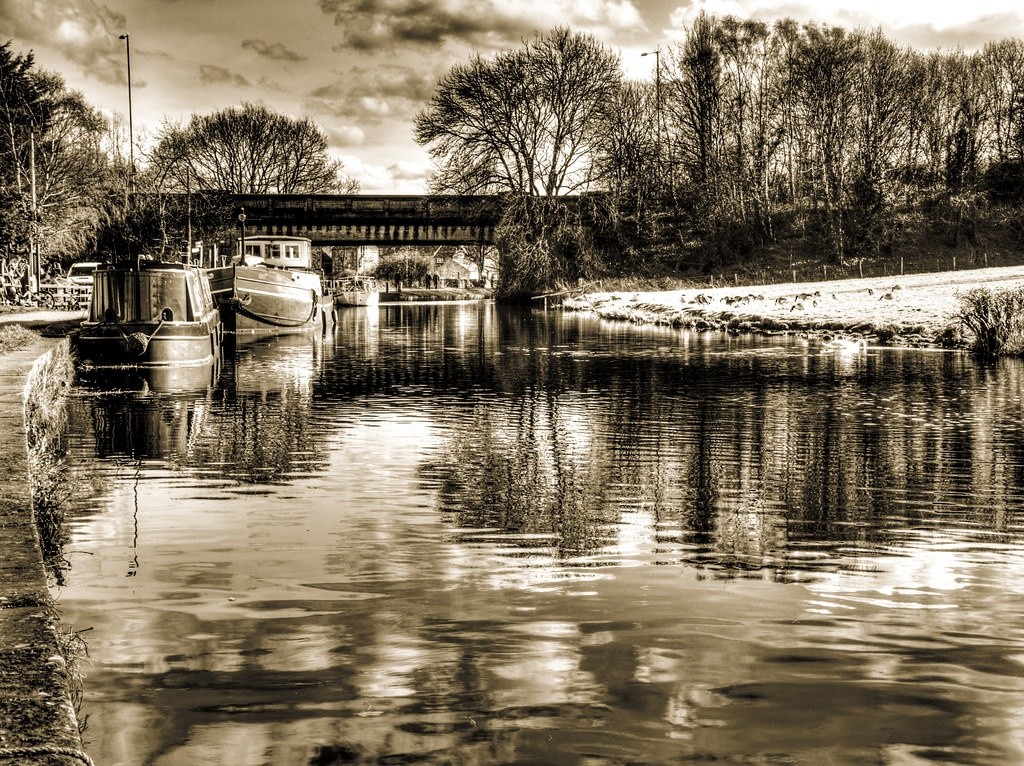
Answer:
[
  {"left": 427, "top": 271, "right": 431, "bottom": 275},
  {"left": 434, "top": 271, "right": 438, "bottom": 275}
]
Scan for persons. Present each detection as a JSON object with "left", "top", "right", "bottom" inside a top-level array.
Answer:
[{"left": 393, "top": 268, "right": 440, "bottom": 290}]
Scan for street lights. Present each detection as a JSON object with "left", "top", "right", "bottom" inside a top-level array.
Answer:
[{"left": 118, "top": 30, "right": 134, "bottom": 187}]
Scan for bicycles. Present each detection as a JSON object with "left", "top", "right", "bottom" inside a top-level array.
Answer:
[{"left": 5, "top": 282, "right": 55, "bottom": 310}]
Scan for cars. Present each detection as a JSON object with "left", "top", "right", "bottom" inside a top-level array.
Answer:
[{"left": 67, "top": 261, "right": 115, "bottom": 305}]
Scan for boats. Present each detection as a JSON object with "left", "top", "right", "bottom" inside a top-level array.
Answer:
[
  {"left": 194, "top": 206, "right": 334, "bottom": 347},
  {"left": 335, "top": 271, "right": 381, "bottom": 307},
  {"left": 74, "top": 254, "right": 224, "bottom": 367}
]
